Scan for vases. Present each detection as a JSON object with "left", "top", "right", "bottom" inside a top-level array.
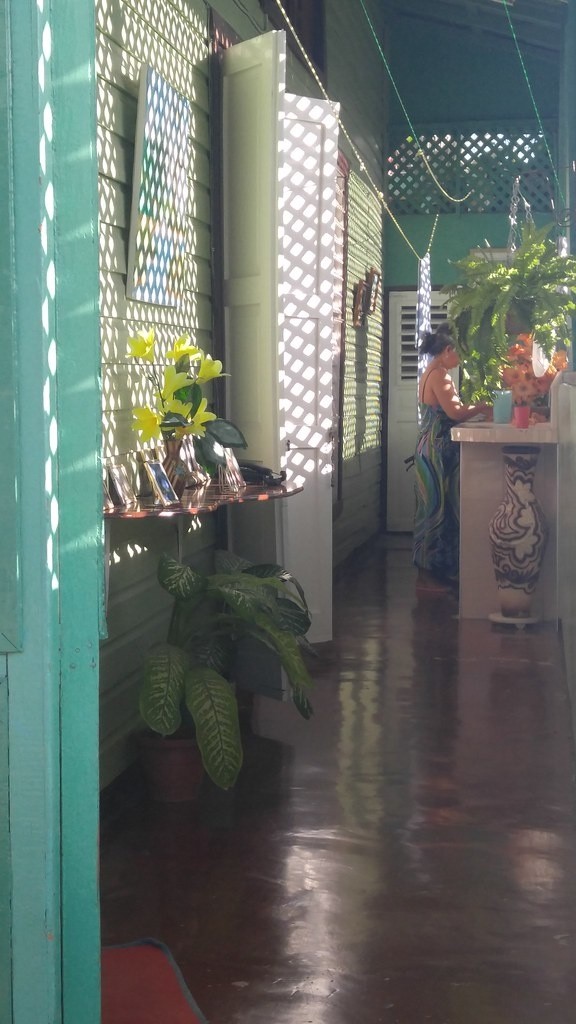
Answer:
[
  {"left": 487, "top": 444, "right": 548, "bottom": 618},
  {"left": 161, "top": 436, "right": 202, "bottom": 489}
]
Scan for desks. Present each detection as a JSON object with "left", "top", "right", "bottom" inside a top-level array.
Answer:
[
  {"left": 451, "top": 412, "right": 557, "bottom": 624},
  {"left": 102, "top": 481, "right": 305, "bottom": 520}
]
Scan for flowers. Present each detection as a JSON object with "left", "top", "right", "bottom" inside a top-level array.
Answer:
[{"left": 126, "top": 329, "right": 232, "bottom": 446}]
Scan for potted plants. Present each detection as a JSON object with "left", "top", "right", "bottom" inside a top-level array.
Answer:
[
  {"left": 438, "top": 220, "right": 576, "bottom": 408},
  {"left": 136, "top": 550, "right": 315, "bottom": 803}
]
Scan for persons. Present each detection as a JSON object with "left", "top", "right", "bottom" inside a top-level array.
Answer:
[{"left": 412, "top": 322, "right": 492, "bottom": 592}]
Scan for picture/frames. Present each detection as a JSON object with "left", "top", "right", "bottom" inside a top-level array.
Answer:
[
  {"left": 353, "top": 279, "right": 370, "bottom": 328},
  {"left": 366, "top": 267, "right": 380, "bottom": 313},
  {"left": 125, "top": 60, "right": 190, "bottom": 308},
  {"left": 109, "top": 465, "right": 137, "bottom": 505},
  {"left": 143, "top": 459, "right": 180, "bottom": 507}
]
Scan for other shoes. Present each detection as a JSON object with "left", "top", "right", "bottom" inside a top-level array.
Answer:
[
  {"left": 430, "top": 562, "right": 459, "bottom": 580},
  {"left": 414, "top": 577, "right": 452, "bottom": 592}
]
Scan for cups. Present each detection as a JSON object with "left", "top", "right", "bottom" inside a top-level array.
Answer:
[
  {"left": 513, "top": 405, "right": 531, "bottom": 428},
  {"left": 491, "top": 390, "right": 513, "bottom": 424}
]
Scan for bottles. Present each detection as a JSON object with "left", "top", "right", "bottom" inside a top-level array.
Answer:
[{"left": 487, "top": 446, "right": 550, "bottom": 618}]
